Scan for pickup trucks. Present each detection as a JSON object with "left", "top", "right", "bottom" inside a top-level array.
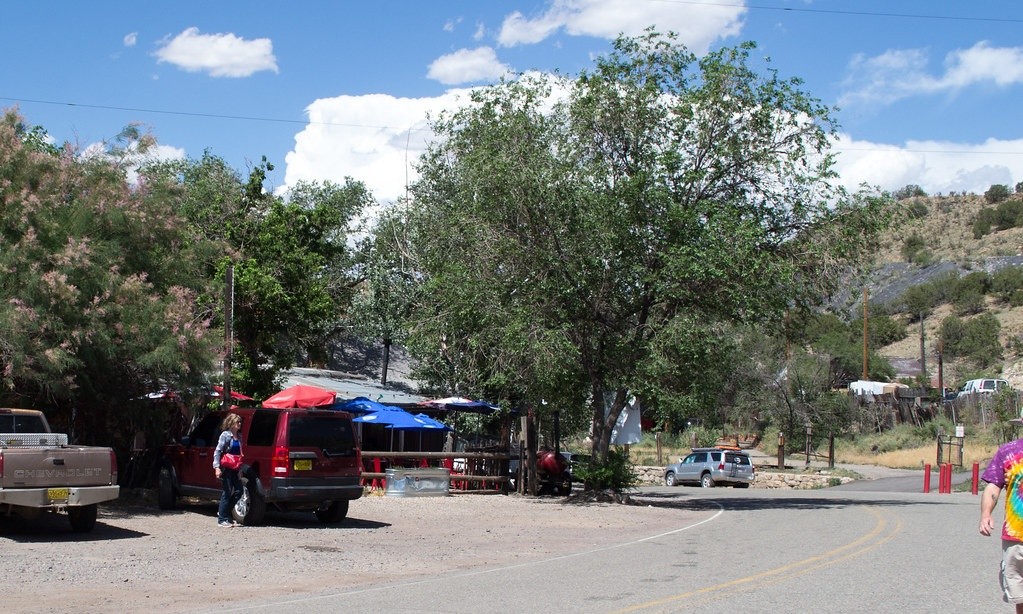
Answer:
[{"left": 0, "top": 408, "right": 121, "bottom": 533}]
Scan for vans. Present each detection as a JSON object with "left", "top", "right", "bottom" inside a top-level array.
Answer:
[
  {"left": 956, "top": 378, "right": 1007, "bottom": 400},
  {"left": 561, "top": 451, "right": 593, "bottom": 483},
  {"left": 157, "top": 407, "right": 364, "bottom": 528}
]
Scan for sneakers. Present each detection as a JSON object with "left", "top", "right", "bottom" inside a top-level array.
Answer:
[
  {"left": 229, "top": 520, "right": 243, "bottom": 527},
  {"left": 218, "top": 520, "right": 232, "bottom": 527}
]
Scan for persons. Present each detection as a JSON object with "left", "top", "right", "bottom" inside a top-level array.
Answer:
[
  {"left": 979, "top": 406, "right": 1023, "bottom": 614},
  {"left": 213, "top": 414, "right": 245, "bottom": 528}
]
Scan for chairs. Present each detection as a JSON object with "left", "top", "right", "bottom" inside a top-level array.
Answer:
[{"left": 370, "top": 456, "right": 468, "bottom": 490}]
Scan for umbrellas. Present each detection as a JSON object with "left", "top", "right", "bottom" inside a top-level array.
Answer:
[
  {"left": 324, "top": 396, "right": 503, "bottom": 452},
  {"left": 261, "top": 385, "right": 337, "bottom": 410},
  {"left": 128, "top": 386, "right": 256, "bottom": 403}
]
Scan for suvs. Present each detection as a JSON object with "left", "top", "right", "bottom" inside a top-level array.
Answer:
[{"left": 665, "top": 445, "right": 755, "bottom": 488}]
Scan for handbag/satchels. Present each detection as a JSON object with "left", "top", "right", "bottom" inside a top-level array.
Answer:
[{"left": 219, "top": 446, "right": 244, "bottom": 470}]
